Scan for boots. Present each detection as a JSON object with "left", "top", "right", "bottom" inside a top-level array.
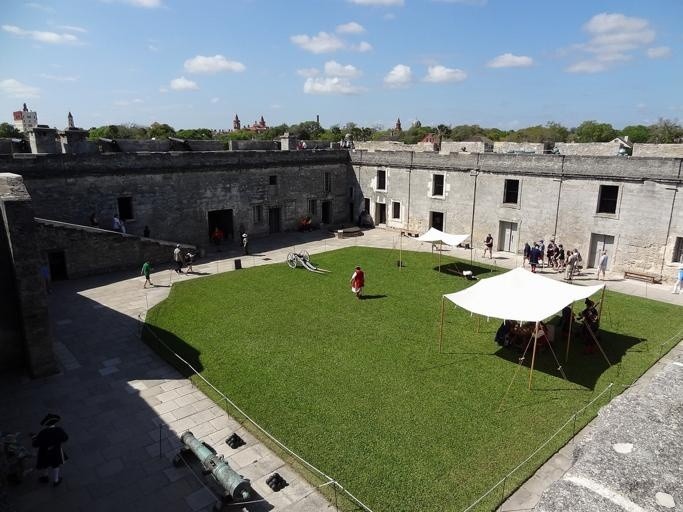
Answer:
[{"left": 39, "top": 476, "right": 62, "bottom": 488}]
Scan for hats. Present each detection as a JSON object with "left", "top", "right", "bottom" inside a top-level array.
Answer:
[{"left": 40, "top": 413, "right": 61, "bottom": 426}]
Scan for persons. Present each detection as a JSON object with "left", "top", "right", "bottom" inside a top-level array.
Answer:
[
  {"left": 596, "top": 250, "right": 608, "bottom": 280},
  {"left": 140, "top": 259, "right": 153, "bottom": 288},
  {"left": 300, "top": 215, "right": 312, "bottom": 232},
  {"left": 351, "top": 267, "right": 364, "bottom": 299},
  {"left": 495, "top": 300, "right": 599, "bottom": 353},
  {"left": 242, "top": 233, "right": 249, "bottom": 255},
  {"left": 482, "top": 233, "right": 493, "bottom": 259},
  {"left": 40, "top": 262, "right": 51, "bottom": 294},
  {"left": 144, "top": 225, "right": 151, "bottom": 238},
  {"left": 297, "top": 140, "right": 307, "bottom": 151},
  {"left": 672, "top": 268, "right": 683, "bottom": 295},
  {"left": 30, "top": 414, "right": 69, "bottom": 486},
  {"left": 174, "top": 243, "right": 196, "bottom": 273},
  {"left": 522, "top": 239, "right": 583, "bottom": 280},
  {"left": 211, "top": 228, "right": 224, "bottom": 252},
  {"left": 112, "top": 214, "right": 127, "bottom": 233}
]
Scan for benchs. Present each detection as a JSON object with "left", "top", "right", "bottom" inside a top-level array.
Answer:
[{"left": 624, "top": 271, "right": 654, "bottom": 283}]
